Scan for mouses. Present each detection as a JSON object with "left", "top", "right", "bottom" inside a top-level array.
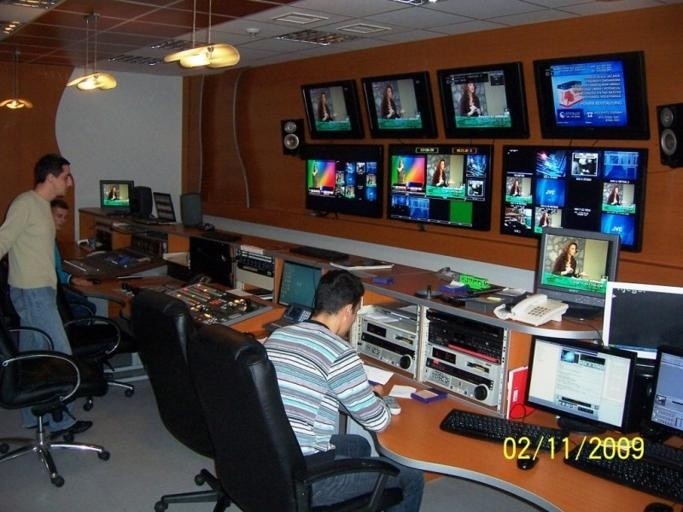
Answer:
[
  {"left": 641, "top": 502, "right": 674, "bottom": 512},
  {"left": 203, "top": 223, "right": 215, "bottom": 232},
  {"left": 518, "top": 453, "right": 539, "bottom": 470}
]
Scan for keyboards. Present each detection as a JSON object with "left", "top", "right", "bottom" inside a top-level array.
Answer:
[
  {"left": 439, "top": 408, "right": 569, "bottom": 454},
  {"left": 564, "top": 439, "right": 683, "bottom": 504},
  {"left": 202, "top": 233, "right": 241, "bottom": 243},
  {"left": 289, "top": 246, "right": 350, "bottom": 263}
]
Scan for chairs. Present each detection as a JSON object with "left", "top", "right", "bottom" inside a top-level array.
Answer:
[
  {"left": 133, "top": 287, "right": 231, "bottom": 512},
  {"left": 188, "top": 325, "right": 403, "bottom": 512},
  {"left": 0, "top": 258, "right": 134, "bottom": 486}
]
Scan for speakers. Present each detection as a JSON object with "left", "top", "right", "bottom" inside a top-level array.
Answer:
[
  {"left": 655, "top": 102, "right": 683, "bottom": 169},
  {"left": 134, "top": 186, "right": 153, "bottom": 221},
  {"left": 280, "top": 118, "right": 305, "bottom": 156},
  {"left": 179, "top": 192, "right": 203, "bottom": 228}
]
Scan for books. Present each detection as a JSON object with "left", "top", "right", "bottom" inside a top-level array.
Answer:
[{"left": 364, "top": 362, "right": 395, "bottom": 386}]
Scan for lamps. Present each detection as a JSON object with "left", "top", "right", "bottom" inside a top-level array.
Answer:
[
  {"left": 0, "top": 51, "right": 33, "bottom": 109},
  {"left": 163, "top": 0, "right": 241, "bottom": 69},
  {"left": 66, "top": 12, "right": 117, "bottom": 90}
]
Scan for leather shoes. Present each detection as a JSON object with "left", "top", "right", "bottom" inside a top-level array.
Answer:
[{"left": 51, "top": 420, "right": 91, "bottom": 435}]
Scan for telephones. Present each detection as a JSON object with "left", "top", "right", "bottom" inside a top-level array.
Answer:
[{"left": 510, "top": 294, "right": 570, "bottom": 327}]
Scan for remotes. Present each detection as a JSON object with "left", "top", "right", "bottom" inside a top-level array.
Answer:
[{"left": 382, "top": 395, "right": 401, "bottom": 416}]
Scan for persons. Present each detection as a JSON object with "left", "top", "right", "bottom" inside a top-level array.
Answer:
[
  {"left": 263, "top": 269, "right": 426, "bottom": 511},
  {"left": 0, "top": 153, "right": 93, "bottom": 436},
  {"left": 510, "top": 179, "right": 521, "bottom": 196},
  {"left": 460, "top": 82, "right": 483, "bottom": 117},
  {"left": 108, "top": 186, "right": 118, "bottom": 200},
  {"left": 540, "top": 212, "right": 549, "bottom": 227},
  {"left": 50, "top": 198, "right": 95, "bottom": 316},
  {"left": 317, "top": 92, "right": 334, "bottom": 121},
  {"left": 312, "top": 160, "right": 374, "bottom": 198},
  {"left": 607, "top": 186, "right": 620, "bottom": 205},
  {"left": 551, "top": 243, "right": 588, "bottom": 279},
  {"left": 380, "top": 86, "right": 400, "bottom": 119},
  {"left": 397, "top": 158, "right": 482, "bottom": 208}
]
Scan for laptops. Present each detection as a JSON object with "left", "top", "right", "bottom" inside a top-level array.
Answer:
[{"left": 136, "top": 191, "right": 177, "bottom": 224}]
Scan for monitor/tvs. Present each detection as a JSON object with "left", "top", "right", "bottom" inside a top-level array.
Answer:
[
  {"left": 524, "top": 334, "right": 638, "bottom": 435},
  {"left": 361, "top": 71, "right": 438, "bottom": 140},
  {"left": 532, "top": 51, "right": 651, "bottom": 141},
  {"left": 301, "top": 79, "right": 364, "bottom": 141},
  {"left": 100, "top": 179, "right": 134, "bottom": 217},
  {"left": 533, "top": 227, "right": 621, "bottom": 320},
  {"left": 436, "top": 61, "right": 531, "bottom": 140},
  {"left": 499, "top": 145, "right": 650, "bottom": 254},
  {"left": 600, "top": 280, "right": 683, "bottom": 357},
  {"left": 385, "top": 143, "right": 493, "bottom": 231},
  {"left": 277, "top": 259, "right": 324, "bottom": 315},
  {"left": 303, "top": 143, "right": 384, "bottom": 220},
  {"left": 188, "top": 236, "right": 235, "bottom": 289}
]
